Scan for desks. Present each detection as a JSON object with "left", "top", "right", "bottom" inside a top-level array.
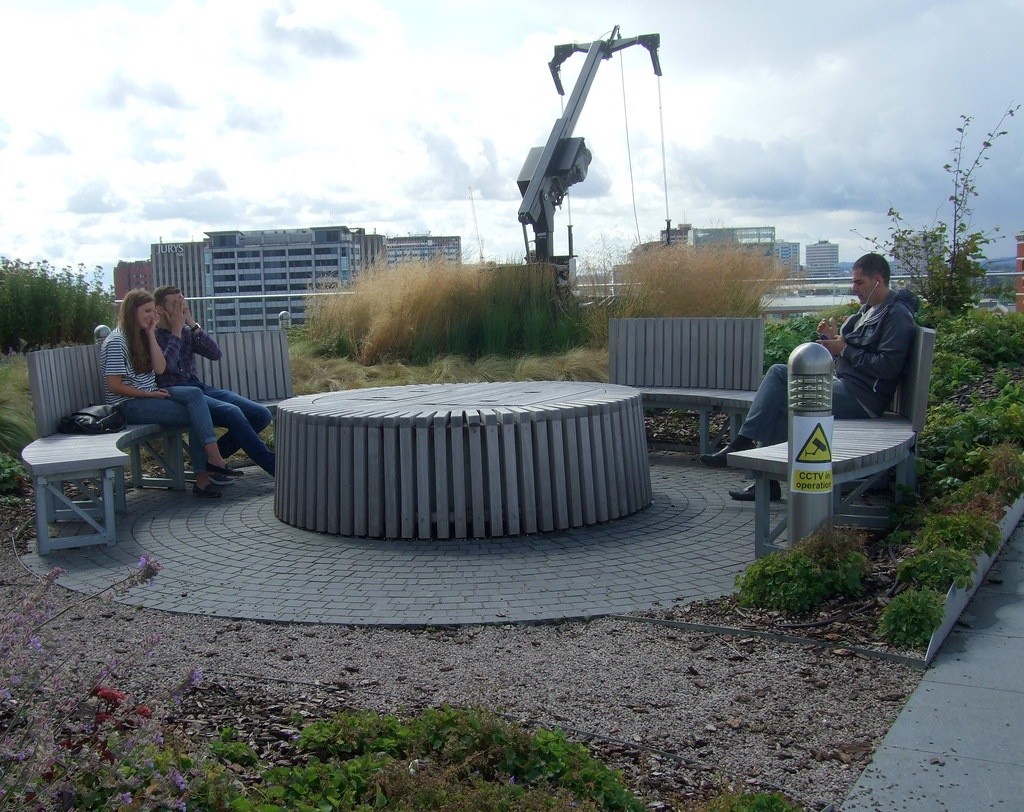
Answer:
[{"left": 275, "top": 380, "right": 655, "bottom": 541}]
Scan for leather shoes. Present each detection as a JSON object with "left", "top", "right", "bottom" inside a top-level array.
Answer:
[
  {"left": 729, "top": 480, "right": 781, "bottom": 501},
  {"left": 700, "top": 438, "right": 756, "bottom": 467}
]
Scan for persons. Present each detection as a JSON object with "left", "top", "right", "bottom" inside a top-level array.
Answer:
[
  {"left": 100, "top": 289, "right": 245, "bottom": 499},
  {"left": 152, "top": 286, "right": 275, "bottom": 486},
  {"left": 697, "top": 253, "right": 922, "bottom": 502}
]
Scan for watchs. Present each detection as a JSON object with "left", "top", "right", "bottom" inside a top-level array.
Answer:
[{"left": 191, "top": 323, "right": 201, "bottom": 333}]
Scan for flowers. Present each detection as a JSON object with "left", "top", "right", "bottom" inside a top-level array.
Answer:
[{"left": 0, "top": 553, "right": 204, "bottom": 812}]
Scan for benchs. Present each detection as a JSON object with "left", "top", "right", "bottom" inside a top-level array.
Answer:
[
  {"left": 607, "top": 318, "right": 937, "bottom": 562},
  {"left": 22, "top": 330, "right": 295, "bottom": 556}
]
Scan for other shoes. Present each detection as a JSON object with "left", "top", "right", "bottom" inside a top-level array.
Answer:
[
  {"left": 207, "top": 473, "right": 233, "bottom": 485},
  {"left": 192, "top": 483, "right": 223, "bottom": 498},
  {"left": 205, "top": 462, "right": 244, "bottom": 476}
]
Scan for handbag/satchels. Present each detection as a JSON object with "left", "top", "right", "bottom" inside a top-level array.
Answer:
[{"left": 58, "top": 402, "right": 126, "bottom": 434}]
